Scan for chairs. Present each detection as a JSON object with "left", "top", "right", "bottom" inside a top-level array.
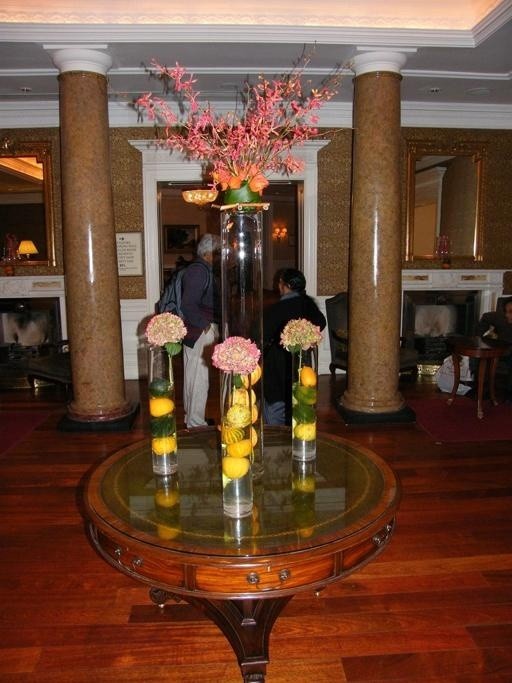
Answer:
[
  {"left": 470, "top": 296, "right": 512, "bottom": 401},
  {"left": 324, "top": 291, "right": 420, "bottom": 386}
]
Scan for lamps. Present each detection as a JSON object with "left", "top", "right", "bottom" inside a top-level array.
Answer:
[{"left": 15, "top": 240, "right": 39, "bottom": 259}]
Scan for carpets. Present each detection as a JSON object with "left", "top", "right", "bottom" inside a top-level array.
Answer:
[{"left": 398, "top": 386, "right": 511, "bottom": 443}]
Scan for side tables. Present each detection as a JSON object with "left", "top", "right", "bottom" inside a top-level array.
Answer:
[{"left": 444, "top": 335, "right": 510, "bottom": 421}]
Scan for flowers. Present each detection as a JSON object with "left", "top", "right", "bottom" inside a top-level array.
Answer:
[
  {"left": 210, "top": 337, "right": 261, "bottom": 406},
  {"left": 144, "top": 312, "right": 189, "bottom": 356},
  {"left": 134, "top": 40, "right": 356, "bottom": 192},
  {"left": 279, "top": 314, "right": 323, "bottom": 382}
]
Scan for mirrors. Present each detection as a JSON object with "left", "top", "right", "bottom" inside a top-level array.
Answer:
[
  {"left": 0, "top": 138, "right": 55, "bottom": 270},
  {"left": 404, "top": 138, "right": 490, "bottom": 263}
]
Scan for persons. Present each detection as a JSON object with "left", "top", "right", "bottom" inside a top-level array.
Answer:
[
  {"left": 177, "top": 232, "right": 231, "bottom": 429},
  {"left": 260, "top": 267, "right": 327, "bottom": 427},
  {"left": 481, "top": 300, "right": 511, "bottom": 344}
]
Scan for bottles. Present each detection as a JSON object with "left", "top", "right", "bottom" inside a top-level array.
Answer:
[{"left": 145, "top": 345, "right": 318, "bottom": 519}]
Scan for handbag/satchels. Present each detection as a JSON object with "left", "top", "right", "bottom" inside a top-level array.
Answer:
[{"left": 435, "top": 355, "right": 472, "bottom": 396}]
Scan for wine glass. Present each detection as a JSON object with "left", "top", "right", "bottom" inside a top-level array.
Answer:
[
  {"left": 1, "top": 246, "right": 23, "bottom": 261},
  {"left": 431, "top": 236, "right": 457, "bottom": 266}
]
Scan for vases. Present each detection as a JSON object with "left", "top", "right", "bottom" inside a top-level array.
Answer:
[
  {"left": 289, "top": 348, "right": 319, "bottom": 477},
  {"left": 224, "top": 181, "right": 259, "bottom": 206},
  {"left": 218, "top": 370, "right": 255, "bottom": 518},
  {"left": 148, "top": 344, "right": 178, "bottom": 476}
]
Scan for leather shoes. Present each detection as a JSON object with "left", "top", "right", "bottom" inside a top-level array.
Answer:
[{"left": 184, "top": 417, "right": 215, "bottom": 426}]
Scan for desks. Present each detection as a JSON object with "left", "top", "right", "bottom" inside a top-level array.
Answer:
[{"left": 82, "top": 424, "right": 402, "bottom": 683}]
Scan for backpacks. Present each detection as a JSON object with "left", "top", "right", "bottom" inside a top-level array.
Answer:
[{"left": 158, "top": 263, "right": 210, "bottom": 321}]
